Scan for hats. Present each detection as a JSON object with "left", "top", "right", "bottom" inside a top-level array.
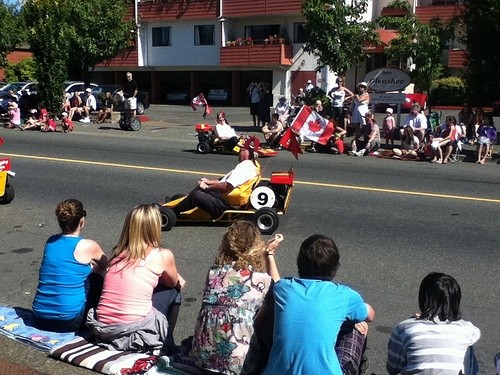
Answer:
[
  {"left": 217, "top": 112, "right": 225, "bottom": 120},
  {"left": 365, "top": 112, "right": 376, "bottom": 122},
  {"left": 357, "top": 82, "right": 368, "bottom": 86},
  {"left": 237, "top": 135, "right": 259, "bottom": 152},
  {"left": 86, "top": 88, "right": 92, "bottom": 93},
  {"left": 10, "top": 102, "right": 18, "bottom": 109},
  {"left": 386, "top": 108, "right": 393, "bottom": 114}
]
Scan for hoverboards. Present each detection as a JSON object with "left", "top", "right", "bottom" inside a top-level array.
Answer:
[{"left": 119, "top": 94, "right": 141, "bottom": 131}]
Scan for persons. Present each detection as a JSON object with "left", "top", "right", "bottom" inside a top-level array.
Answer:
[
  {"left": 393, "top": 125, "right": 420, "bottom": 159},
  {"left": 248, "top": 78, "right": 347, "bottom": 154},
  {"left": 84, "top": 203, "right": 186, "bottom": 351},
  {"left": 123, "top": 72, "right": 139, "bottom": 117},
  {"left": 399, "top": 103, "right": 427, "bottom": 149},
  {"left": 427, "top": 101, "right": 500, "bottom": 164},
  {"left": 151, "top": 147, "right": 260, "bottom": 220},
  {"left": 191, "top": 220, "right": 285, "bottom": 375},
  {"left": 328, "top": 78, "right": 354, "bottom": 142},
  {"left": 352, "top": 82, "right": 369, "bottom": 138},
  {"left": 4, "top": 88, "right": 113, "bottom": 133},
  {"left": 32, "top": 199, "right": 108, "bottom": 333},
  {"left": 214, "top": 114, "right": 260, "bottom": 150},
  {"left": 386, "top": 272, "right": 482, "bottom": 375},
  {"left": 348, "top": 112, "right": 381, "bottom": 157},
  {"left": 383, "top": 108, "right": 395, "bottom": 149},
  {"left": 262, "top": 235, "right": 375, "bottom": 375}
]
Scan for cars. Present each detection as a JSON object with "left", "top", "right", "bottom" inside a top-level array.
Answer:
[
  {"left": 166, "top": 89, "right": 188, "bottom": 100},
  {"left": 62, "top": 82, "right": 103, "bottom": 106},
  {"left": 99, "top": 85, "right": 150, "bottom": 115},
  {"left": 377, "top": 84, "right": 428, "bottom": 111},
  {"left": 208, "top": 88, "right": 229, "bottom": 103}
]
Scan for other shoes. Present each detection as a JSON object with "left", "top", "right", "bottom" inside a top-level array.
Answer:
[
  {"left": 79, "top": 118, "right": 84, "bottom": 122},
  {"left": 82, "top": 118, "right": 90, "bottom": 123},
  {"left": 436, "top": 159, "right": 442, "bottom": 164},
  {"left": 443, "top": 160, "right": 448, "bottom": 163},
  {"left": 431, "top": 158, "right": 437, "bottom": 162},
  {"left": 360, "top": 355, "right": 369, "bottom": 373},
  {"left": 347, "top": 151, "right": 357, "bottom": 156},
  {"left": 476, "top": 160, "right": 480, "bottom": 163},
  {"left": 479, "top": 160, "right": 485, "bottom": 164},
  {"left": 356, "top": 152, "right": 363, "bottom": 156}
]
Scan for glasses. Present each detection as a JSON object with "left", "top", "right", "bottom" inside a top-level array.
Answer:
[{"left": 81, "top": 209, "right": 87, "bottom": 218}]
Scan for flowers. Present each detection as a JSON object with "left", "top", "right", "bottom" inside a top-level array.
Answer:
[
  {"left": 246, "top": 36, "right": 252, "bottom": 41},
  {"left": 236, "top": 37, "right": 242, "bottom": 42}
]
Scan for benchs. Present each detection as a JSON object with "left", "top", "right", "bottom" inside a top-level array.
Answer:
[{"left": 66, "top": 110, "right": 111, "bottom": 122}]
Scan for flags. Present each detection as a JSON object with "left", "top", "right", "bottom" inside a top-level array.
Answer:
[
  {"left": 290, "top": 104, "right": 337, "bottom": 145},
  {"left": 190, "top": 93, "right": 207, "bottom": 111}
]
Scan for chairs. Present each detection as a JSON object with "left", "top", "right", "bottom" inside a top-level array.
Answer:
[{"left": 448, "top": 143, "right": 493, "bottom": 164}]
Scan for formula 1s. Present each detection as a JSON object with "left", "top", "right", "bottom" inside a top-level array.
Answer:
[
  {"left": 160, "top": 166, "right": 294, "bottom": 234},
  {"left": 194, "top": 122, "right": 279, "bottom": 157}
]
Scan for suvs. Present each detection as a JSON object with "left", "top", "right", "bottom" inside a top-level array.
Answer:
[{"left": 0, "top": 82, "right": 45, "bottom": 114}]
